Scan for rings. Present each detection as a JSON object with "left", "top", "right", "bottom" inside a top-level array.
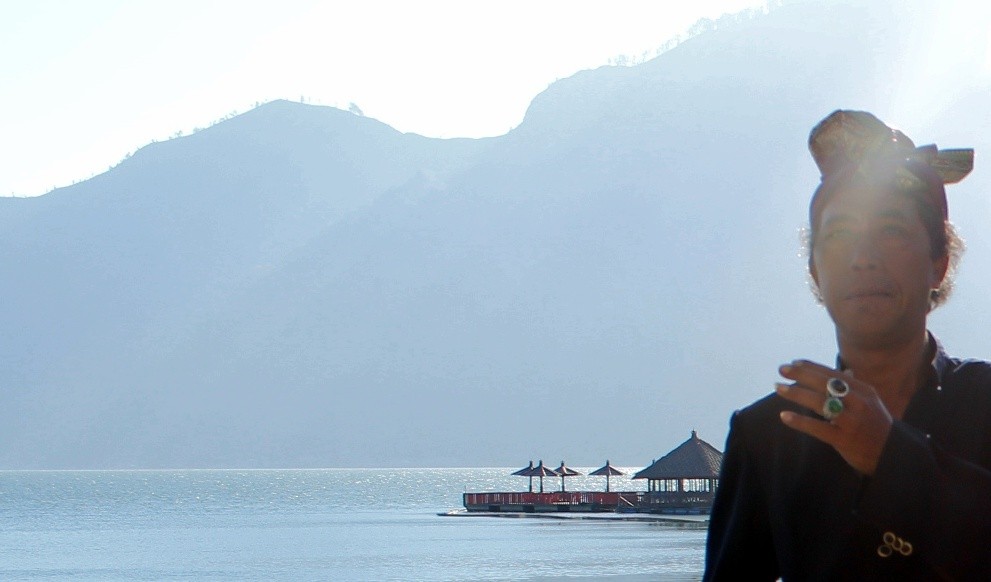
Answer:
[
  {"left": 827, "top": 378, "right": 849, "bottom": 397},
  {"left": 824, "top": 397, "right": 843, "bottom": 419}
]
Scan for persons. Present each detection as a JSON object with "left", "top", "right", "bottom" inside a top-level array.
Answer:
[{"left": 703, "top": 109, "right": 991, "bottom": 582}]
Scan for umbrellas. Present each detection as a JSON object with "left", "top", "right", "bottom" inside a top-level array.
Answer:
[
  {"left": 588, "top": 460, "right": 623, "bottom": 492},
  {"left": 553, "top": 461, "right": 583, "bottom": 491},
  {"left": 511, "top": 461, "right": 536, "bottom": 492},
  {"left": 521, "top": 460, "right": 562, "bottom": 492}
]
